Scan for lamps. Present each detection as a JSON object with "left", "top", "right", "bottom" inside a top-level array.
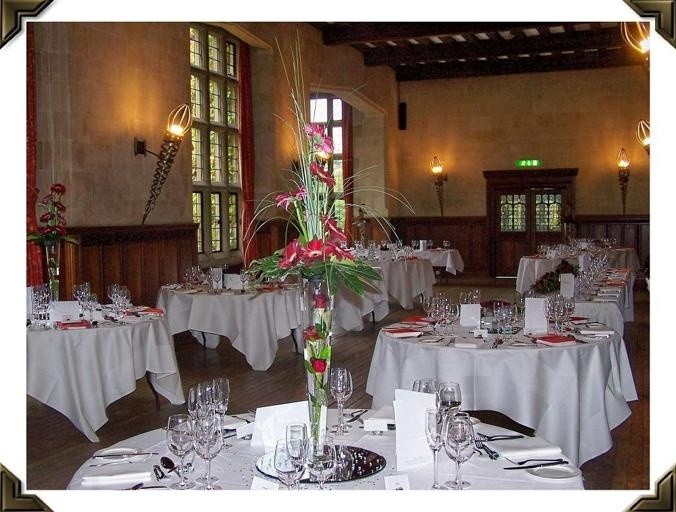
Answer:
[
  {"left": 140, "top": 102, "right": 194, "bottom": 225},
  {"left": 616, "top": 147, "right": 631, "bottom": 214},
  {"left": 430, "top": 155, "right": 448, "bottom": 217},
  {"left": 635, "top": 119, "right": 650, "bottom": 155},
  {"left": 619, "top": 23, "right": 649, "bottom": 73}
]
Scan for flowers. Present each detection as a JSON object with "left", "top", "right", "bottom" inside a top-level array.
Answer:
[
  {"left": 235, "top": 25, "right": 414, "bottom": 450},
  {"left": 27, "top": 183, "right": 81, "bottom": 294}
]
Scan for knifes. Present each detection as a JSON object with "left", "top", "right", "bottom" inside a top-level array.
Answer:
[
  {"left": 347, "top": 408, "right": 367, "bottom": 423},
  {"left": 504, "top": 461, "right": 568, "bottom": 470}
]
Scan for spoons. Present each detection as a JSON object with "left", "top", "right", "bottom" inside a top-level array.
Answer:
[
  {"left": 160, "top": 457, "right": 189, "bottom": 482},
  {"left": 476, "top": 432, "right": 524, "bottom": 440},
  {"left": 232, "top": 415, "right": 250, "bottom": 424}
]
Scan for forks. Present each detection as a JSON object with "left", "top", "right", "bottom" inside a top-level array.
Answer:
[
  {"left": 342, "top": 410, "right": 365, "bottom": 416},
  {"left": 475, "top": 438, "right": 497, "bottom": 463},
  {"left": 504, "top": 453, "right": 563, "bottom": 465}
]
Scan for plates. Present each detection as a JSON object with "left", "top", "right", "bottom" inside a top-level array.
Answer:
[
  {"left": 94, "top": 447, "right": 152, "bottom": 463},
  {"left": 529, "top": 459, "right": 580, "bottom": 478},
  {"left": 383, "top": 328, "right": 422, "bottom": 338}
]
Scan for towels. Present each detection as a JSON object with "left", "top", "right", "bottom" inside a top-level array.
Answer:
[
  {"left": 454, "top": 334, "right": 483, "bottom": 351},
  {"left": 482, "top": 432, "right": 563, "bottom": 467},
  {"left": 577, "top": 323, "right": 616, "bottom": 340},
  {"left": 80, "top": 463, "right": 152, "bottom": 488}
]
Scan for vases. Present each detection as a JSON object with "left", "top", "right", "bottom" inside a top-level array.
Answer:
[
  {"left": 43, "top": 240, "right": 61, "bottom": 313},
  {"left": 256, "top": 277, "right": 386, "bottom": 483}
]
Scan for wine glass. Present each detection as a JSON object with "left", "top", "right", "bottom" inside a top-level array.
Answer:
[
  {"left": 412, "top": 378, "right": 474, "bottom": 491},
  {"left": 423, "top": 236, "right": 617, "bottom": 342},
  {"left": 168, "top": 414, "right": 196, "bottom": 492},
  {"left": 185, "top": 264, "right": 258, "bottom": 293},
  {"left": 274, "top": 422, "right": 335, "bottom": 491},
  {"left": 32, "top": 281, "right": 131, "bottom": 327},
  {"left": 339, "top": 239, "right": 419, "bottom": 261},
  {"left": 187, "top": 378, "right": 231, "bottom": 491},
  {"left": 328, "top": 368, "right": 354, "bottom": 435}
]
